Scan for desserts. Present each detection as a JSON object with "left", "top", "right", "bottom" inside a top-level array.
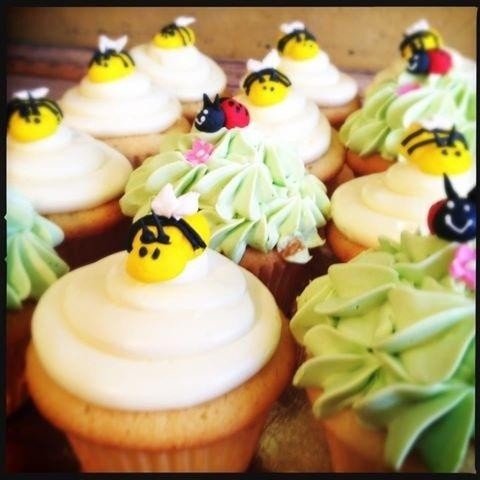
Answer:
[{"left": 5, "top": 15, "right": 477, "bottom": 474}]
